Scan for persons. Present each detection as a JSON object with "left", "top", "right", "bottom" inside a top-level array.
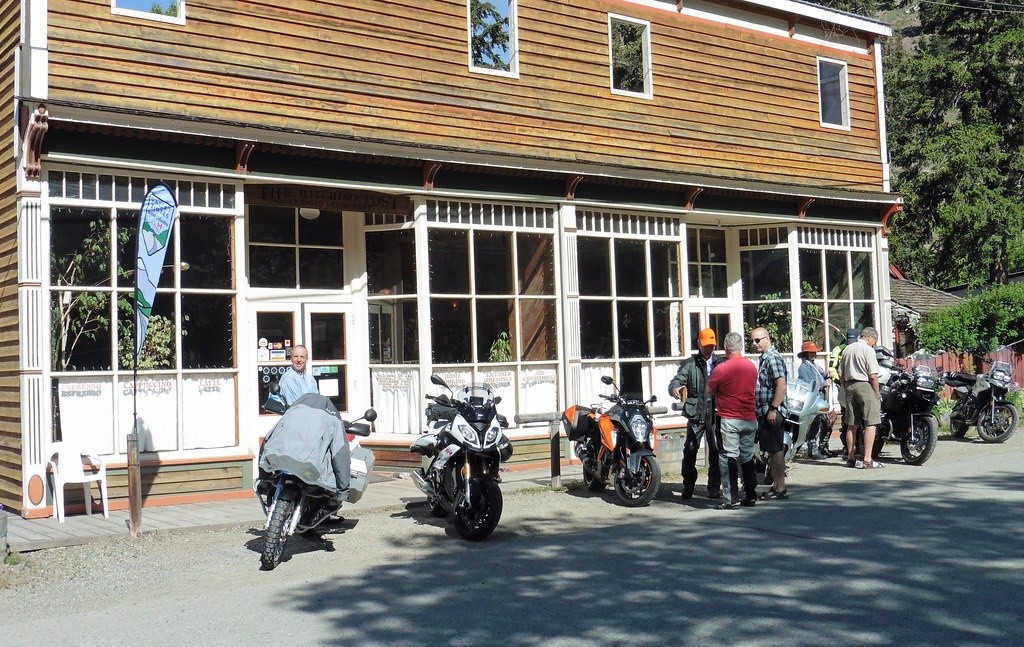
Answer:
[
  {"left": 751, "top": 327, "right": 789, "bottom": 501},
  {"left": 668, "top": 327, "right": 726, "bottom": 500},
  {"left": 828, "top": 327, "right": 886, "bottom": 469},
  {"left": 797, "top": 341, "right": 839, "bottom": 460},
  {"left": 279, "top": 345, "right": 318, "bottom": 405},
  {"left": 706, "top": 333, "right": 758, "bottom": 510}
]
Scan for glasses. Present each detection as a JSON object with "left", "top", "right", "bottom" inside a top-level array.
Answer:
[{"left": 751, "top": 337, "right": 766, "bottom": 343}]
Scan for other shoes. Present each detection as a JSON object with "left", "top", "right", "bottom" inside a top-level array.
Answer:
[
  {"left": 740, "top": 499, "right": 755, "bottom": 507},
  {"left": 821, "top": 450, "right": 838, "bottom": 457},
  {"left": 719, "top": 503, "right": 740, "bottom": 509},
  {"left": 709, "top": 487, "right": 720, "bottom": 498},
  {"left": 810, "top": 454, "right": 827, "bottom": 459}
]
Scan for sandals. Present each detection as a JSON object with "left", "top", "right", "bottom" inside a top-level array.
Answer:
[
  {"left": 846, "top": 458, "right": 856, "bottom": 467},
  {"left": 863, "top": 458, "right": 886, "bottom": 468},
  {"left": 758, "top": 487, "right": 788, "bottom": 500}
]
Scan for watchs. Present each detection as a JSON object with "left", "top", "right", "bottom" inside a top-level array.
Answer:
[{"left": 770, "top": 405, "right": 778, "bottom": 411}]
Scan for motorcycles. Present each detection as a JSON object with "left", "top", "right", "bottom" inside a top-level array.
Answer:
[
  {"left": 409, "top": 374, "right": 514, "bottom": 542},
  {"left": 254, "top": 382, "right": 378, "bottom": 571},
  {"left": 839, "top": 343, "right": 945, "bottom": 465},
  {"left": 938, "top": 338, "right": 1019, "bottom": 443},
  {"left": 561, "top": 375, "right": 668, "bottom": 507},
  {"left": 755, "top": 362, "right": 833, "bottom": 485}
]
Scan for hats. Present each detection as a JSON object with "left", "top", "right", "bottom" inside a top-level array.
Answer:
[
  {"left": 699, "top": 328, "right": 716, "bottom": 346},
  {"left": 847, "top": 329, "right": 858, "bottom": 344},
  {"left": 797, "top": 342, "right": 823, "bottom": 358}
]
